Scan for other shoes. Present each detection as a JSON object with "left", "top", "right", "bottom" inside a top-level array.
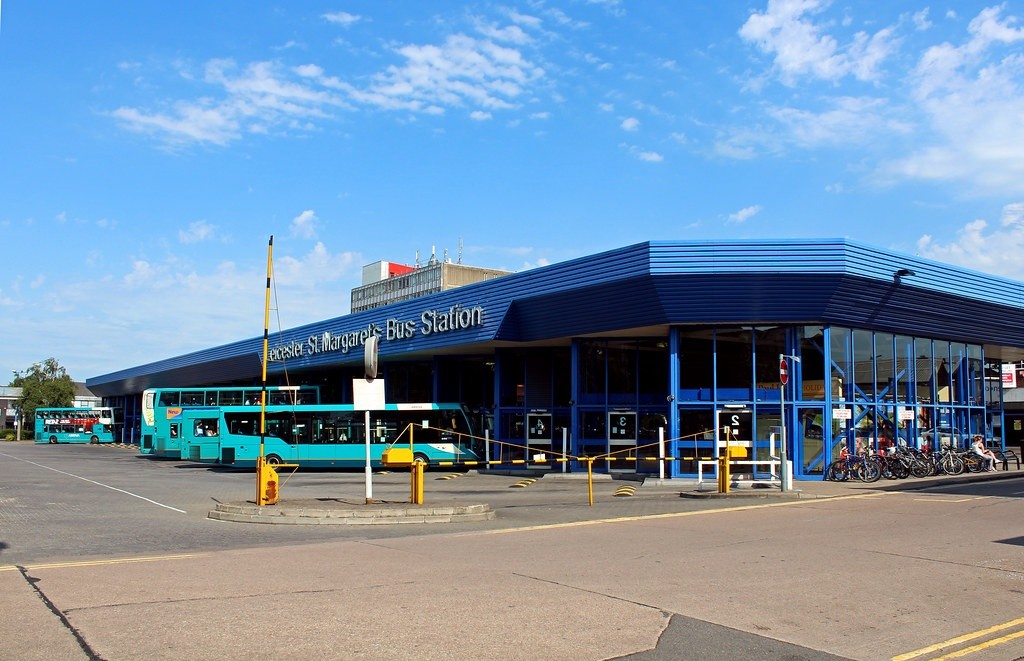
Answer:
[
  {"left": 988, "top": 468, "right": 997, "bottom": 472},
  {"left": 995, "top": 460, "right": 1003, "bottom": 463}
]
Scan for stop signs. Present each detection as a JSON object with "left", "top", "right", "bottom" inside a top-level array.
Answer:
[{"left": 780, "top": 359, "right": 789, "bottom": 384}]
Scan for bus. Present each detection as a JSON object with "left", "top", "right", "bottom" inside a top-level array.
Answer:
[
  {"left": 34, "top": 406, "right": 124, "bottom": 445},
  {"left": 180, "top": 401, "right": 495, "bottom": 473},
  {"left": 139, "top": 385, "right": 320, "bottom": 458}
]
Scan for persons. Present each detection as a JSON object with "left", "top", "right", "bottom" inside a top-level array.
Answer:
[
  {"left": 256, "top": 397, "right": 262, "bottom": 405},
  {"left": 922, "top": 436, "right": 933, "bottom": 453},
  {"left": 330, "top": 430, "right": 334, "bottom": 438},
  {"left": 196, "top": 422, "right": 216, "bottom": 436},
  {"left": 972, "top": 436, "right": 1003, "bottom": 473}
]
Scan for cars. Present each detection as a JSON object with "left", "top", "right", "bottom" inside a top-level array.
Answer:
[{"left": 919, "top": 425, "right": 1003, "bottom": 450}]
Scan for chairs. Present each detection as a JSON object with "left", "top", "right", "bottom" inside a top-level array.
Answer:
[{"left": 985, "top": 450, "right": 1020, "bottom": 472}]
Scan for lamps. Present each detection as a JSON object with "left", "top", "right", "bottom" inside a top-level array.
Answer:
[{"left": 893, "top": 269, "right": 915, "bottom": 276}]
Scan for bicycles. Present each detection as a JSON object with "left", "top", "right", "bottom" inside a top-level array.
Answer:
[{"left": 828, "top": 437, "right": 988, "bottom": 484}]
